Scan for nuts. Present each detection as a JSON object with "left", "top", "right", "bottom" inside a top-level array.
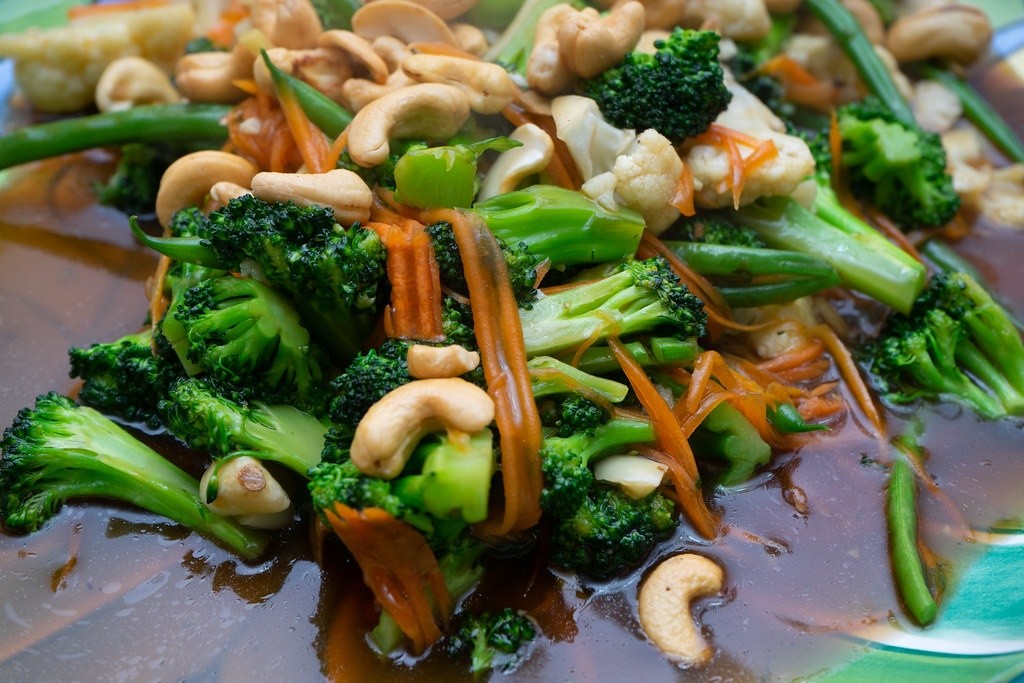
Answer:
[{"left": 92, "top": 0, "right": 991, "bottom": 666}]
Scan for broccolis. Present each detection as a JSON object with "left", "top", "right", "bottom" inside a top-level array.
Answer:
[{"left": 0, "top": 28, "right": 1024, "bottom": 679}]
